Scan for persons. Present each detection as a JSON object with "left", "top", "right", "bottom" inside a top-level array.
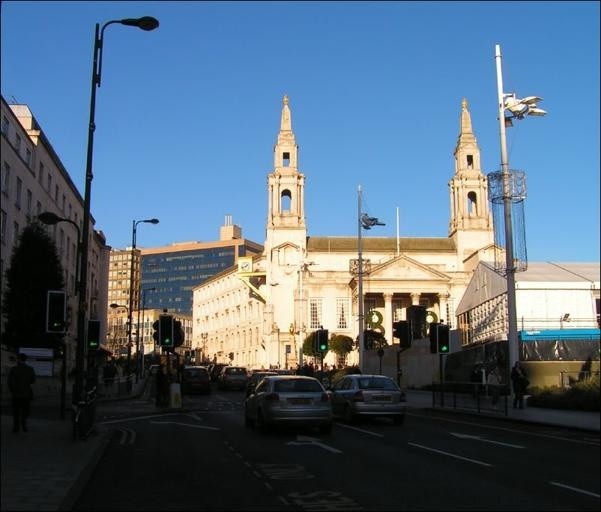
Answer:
[{"left": 8, "top": 354, "right": 36, "bottom": 431}]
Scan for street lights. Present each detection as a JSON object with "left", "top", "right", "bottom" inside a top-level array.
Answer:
[
  {"left": 108, "top": 304, "right": 128, "bottom": 322},
  {"left": 37, "top": 14, "right": 160, "bottom": 434},
  {"left": 127, "top": 217, "right": 159, "bottom": 378},
  {"left": 142, "top": 287, "right": 156, "bottom": 375}
]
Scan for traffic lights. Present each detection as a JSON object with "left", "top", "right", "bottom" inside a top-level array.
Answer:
[
  {"left": 318, "top": 329, "right": 329, "bottom": 352},
  {"left": 87, "top": 320, "right": 101, "bottom": 351},
  {"left": 436, "top": 324, "right": 450, "bottom": 355},
  {"left": 153, "top": 313, "right": 183, "bottom": 346},
  {"left": 392, "top": 321, "right": 412, "bottom": 349},
  {"left": 47, "top": 289, "right": 67, "bottom": 335}
]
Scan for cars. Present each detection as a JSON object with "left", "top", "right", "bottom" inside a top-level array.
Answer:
[
  {"left": 244, "top": 375, "right": 335, "bottom": 432},
  {"left": 148, "top": 362, "right": 281, "bottom": 395},
  {"left": 324, "top": 374, "right": 408, "bottom": 424}
]
